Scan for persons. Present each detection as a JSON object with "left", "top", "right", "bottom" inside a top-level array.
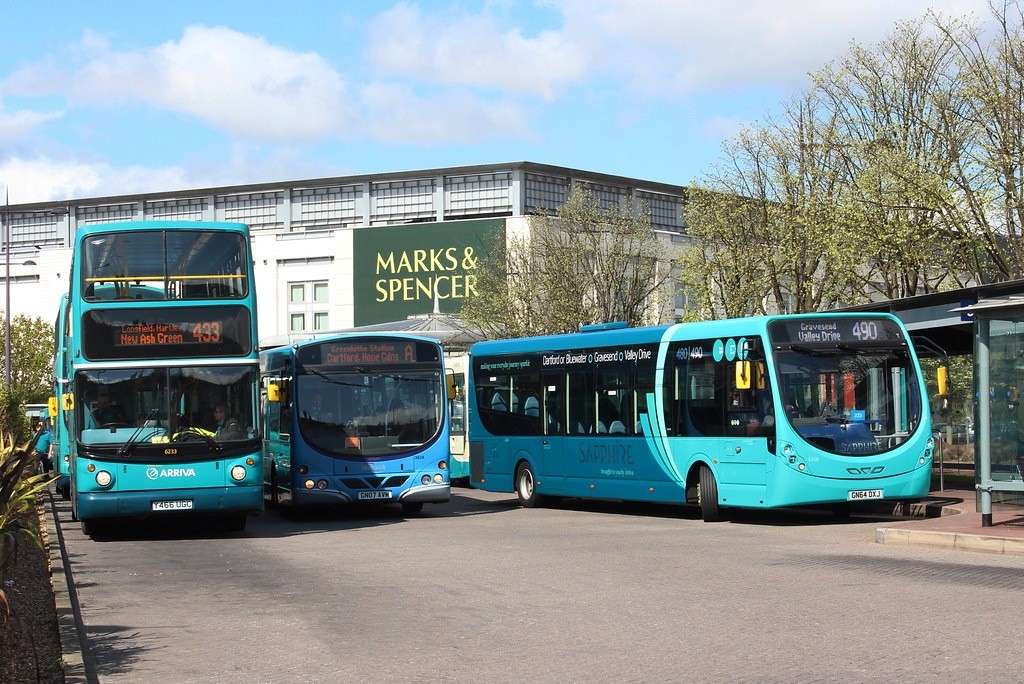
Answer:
[
  {"left": 212, "top": 402, "right": 246, "bottom": 441},
  {"left": 33, "top": 420, "right": 54, "bottom": 483},
  {"left": 89, "top": 384, "right": 127, "bottom": 429}
]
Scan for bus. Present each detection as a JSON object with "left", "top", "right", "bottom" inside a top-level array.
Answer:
[
  {"left": 438, "top": 312, "right": 955, "bottom": 522},
  {"left": 258, "top": 331, "right": 457, "bottom": 518},
  {"left": 44, "top": 217, "right": 265, "bottom": 535}
]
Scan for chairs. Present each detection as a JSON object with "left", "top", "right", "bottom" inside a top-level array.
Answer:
[{"left": 491, "top": 387, "right": 645, "bottom": 435}]
